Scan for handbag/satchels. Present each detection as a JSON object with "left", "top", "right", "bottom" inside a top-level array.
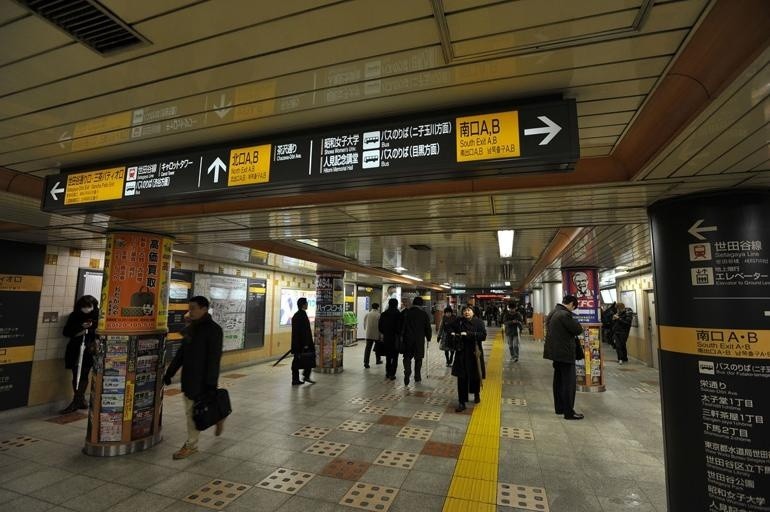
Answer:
[
  {"left": 299, "top": 353, "right": 315, "bottom": 368},
  {"left": 193, "top": 388, "right": 231, "bottom": 430}
]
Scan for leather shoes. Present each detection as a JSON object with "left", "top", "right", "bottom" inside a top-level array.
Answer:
[
  {"left": 292, "top": 377, "right": 315, "bottom": 385},
  {"left": 456, "top": 403, "right": 465, "bottom": 412},
  {"left": 565, "top": 413, "right": 583, "bottom": 419},
  {"left": 174, "top": 448, "right": 198, "bottom": 459},
  {"left": 62, "top": 399, "right": 88, "bottom": 413}
]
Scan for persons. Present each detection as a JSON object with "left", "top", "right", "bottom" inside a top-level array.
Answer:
[
  {"left": 60, "top": 295, "right": 99, "bottom": 414},
  {"left": 572, "top": 272, "right": 595, "bottom": 299},
  {"left": 291, "top": 298, "right": 316, "bottom": 385},
  {"left": 364, "top": 295, "right": 633, "bottom": 420},
  {"left": 164, "top": 296, "right": 223, "bottom": 459}
]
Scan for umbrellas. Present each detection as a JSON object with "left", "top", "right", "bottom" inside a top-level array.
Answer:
[
  {"left": 76, "top": 329, "right": 88, "bottom": 391},
  {"left": 274, "top": 350, "right": 292, "bottom": 367}
]
